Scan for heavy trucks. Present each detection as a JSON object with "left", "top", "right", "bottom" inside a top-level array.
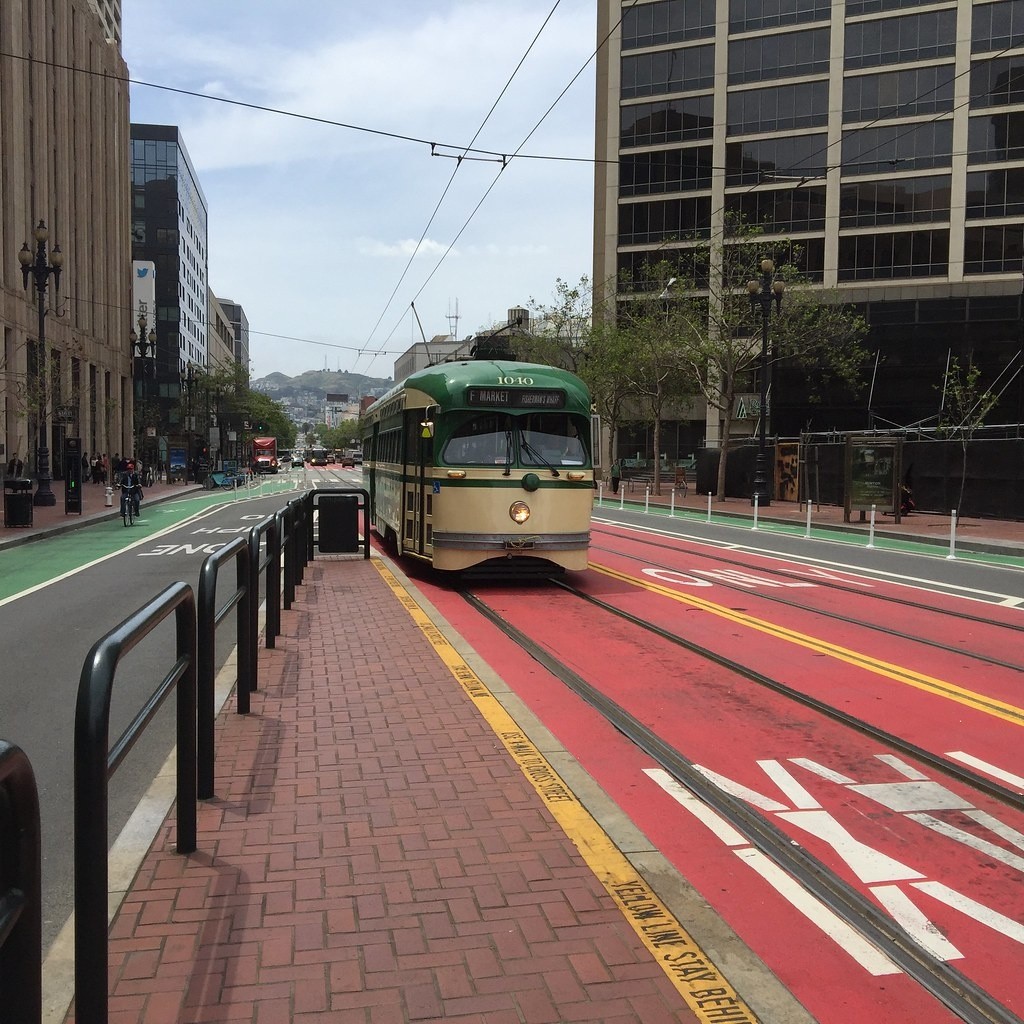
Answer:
[{"left": 251, "top": 436, "right": 278, "bottom": 473}]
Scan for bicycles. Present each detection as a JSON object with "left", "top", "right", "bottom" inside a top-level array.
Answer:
[
  {"left": 204, "top": 473, "right": 236, "bottom": 492},
  {"left": 116, "top": 483, "right": 141, "bottom": 527}
]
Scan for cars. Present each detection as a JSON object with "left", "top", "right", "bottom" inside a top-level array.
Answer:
[
  {"left": 291, "top": 457, "right": 304, "bottom": 468},
  {"left": 342, "top": 458, "right": 354, "bottom": 468},
  {"left": 327, "top": 455, "right": 335, "bottom": 463},
  {"left": 311, "top": 457, "right": 327, "bottom": 465}
]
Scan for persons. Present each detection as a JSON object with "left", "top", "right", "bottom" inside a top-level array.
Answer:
[
  {"left": 112, "top": 453, "right": 164, "bottom": 490},
  {"left": 90, "top": 452, "right": 108, "bottom": 486},
  {"left": 23, "top": 457, "right": 27, "bottom": 465},
  {"left": 7, "top": 453, "right": 23, "bottom": 493},
  {"left": 82, "top": 452, "right": 89, "bottom": 483},
  {"left": 188, "top": 456, "right": 215, "bottom": 484},
  {"left": 165, "top": 459, "right": 171, "bottom": 484},
  {"left": 610, "top": 459, "right": 622, "bottom": 493},
  {"left": 116, "top": 462, "right": 142, "bottom": 516},
  {"left": 546, "top": 435, "right": 573, "bottom": 456}
]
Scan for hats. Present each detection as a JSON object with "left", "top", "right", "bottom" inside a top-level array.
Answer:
[{"left": 126, "top": 464, "right": 134, "bottom": 469}]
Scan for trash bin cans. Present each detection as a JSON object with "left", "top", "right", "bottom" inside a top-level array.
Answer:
[{"left": 3, "top": 478, "right": 34, "bottom": 528}]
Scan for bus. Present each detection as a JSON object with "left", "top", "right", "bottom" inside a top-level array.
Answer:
[
  {"left": 361, "top": 360, "right": 595, "bottom": 588},
  {"left": 277, "top": 449, "right": 296, "bottom": 460},
  {"left": 303, "top": 449, "right": 342, "bottom": 462}
]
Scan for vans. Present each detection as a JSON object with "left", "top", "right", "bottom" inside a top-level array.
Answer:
[{"left": 353, "top": 453, "right": 363, "bottom": 463}]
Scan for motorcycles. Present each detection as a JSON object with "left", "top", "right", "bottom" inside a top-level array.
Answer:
[{"left": 247, "top": 470, "right": 266, "bottom": 479}]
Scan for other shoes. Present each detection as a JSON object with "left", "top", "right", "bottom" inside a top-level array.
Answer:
[
  {"left": 119, "top": 513, "right": 124, "bottom": 516},
  {"left": 135, "top": 513, "right": 140, "bottom": 517},
  {"left": 613, "top": 492, "right": 618, "bottom": 494}
]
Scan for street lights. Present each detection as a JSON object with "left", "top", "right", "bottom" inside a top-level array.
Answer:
[
  {"left": 17, "top": 219, "right": 69, "bottom": 505},
  {"left": 128, "top": 316, "right": 156, "bottom": 486},
  {"left": 747, "top": 253, "right": 786, "bottom": 507},
  {"left": 180, "top": 358, "right": 198, "bottom": 484},
  {"left": 209, "top": 380, "right": 225, "bottom": 472}
]
